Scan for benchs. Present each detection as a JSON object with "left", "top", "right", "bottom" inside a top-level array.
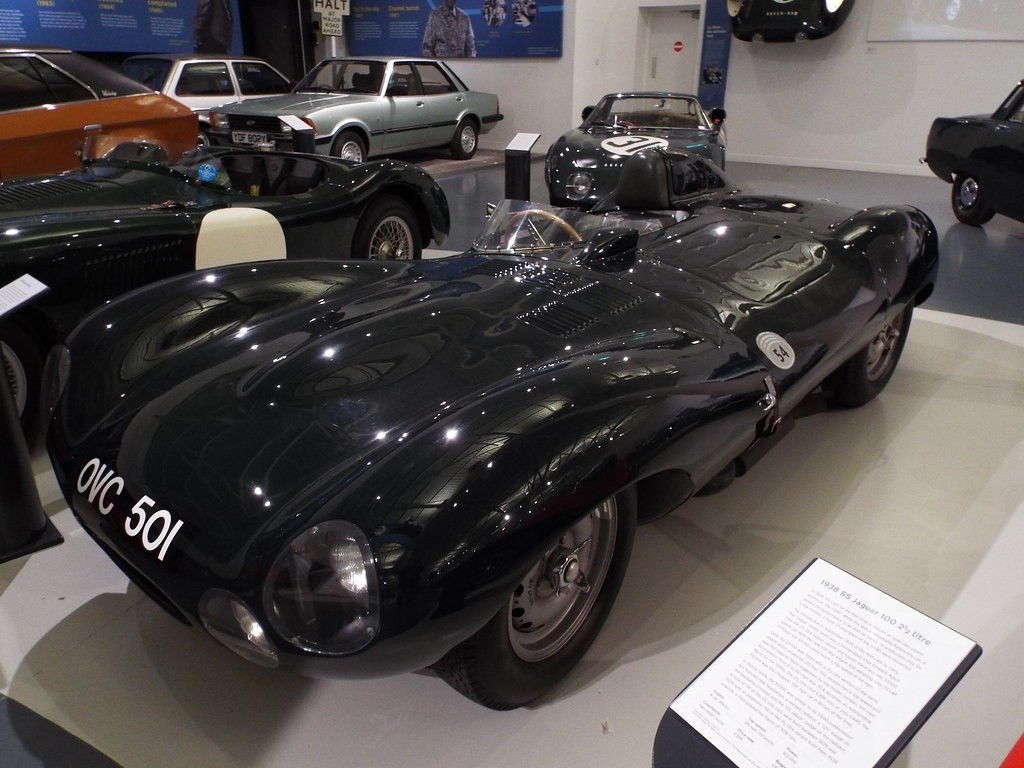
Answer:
[{"left": 611, "top": 110, "right": 700, "bottom": 128}]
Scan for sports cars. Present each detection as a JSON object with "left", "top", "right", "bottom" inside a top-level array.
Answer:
[
  {"left": 41, "top": 148, "right": 941, "bottom": 708},
  {"left": 543, "top": 91, "right": 728, "bottom": 209},
  {"left": 1, "top": 143, "right": 451, "bottom": 447}
]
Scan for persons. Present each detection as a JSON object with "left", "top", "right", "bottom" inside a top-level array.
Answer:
[
  {"left": 483, "top": 0, "right": 507, "bottom": 26},
  {"left": 512, "top": 0, "right": 536, "bottom": 26},
  {"left": 422, "top": 0, "right": 476, "bottom": 58}
]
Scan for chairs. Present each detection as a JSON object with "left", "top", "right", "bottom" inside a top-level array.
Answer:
[
  {"left": 351, "top": 72, "right": 377, "bottom": 94},
  {"left": 390, "top": 72, "right": 416, "bottom": 94}
]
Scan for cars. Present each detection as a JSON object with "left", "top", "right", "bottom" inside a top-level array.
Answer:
[
  {"left": 115, "top": 53, "right": 296, "bottom": 128},
  {"left": 920, "top": 76, "right": 1024, "bottom": 228},
  {"left": 704, "top": 70, "right": 722, "bottom": 84},
  {"left": 1, "top": 43, "right": 211, "bottom": 189},
  {"left": 207, "top": 56, "right": 503, "bottom": 171}
]
